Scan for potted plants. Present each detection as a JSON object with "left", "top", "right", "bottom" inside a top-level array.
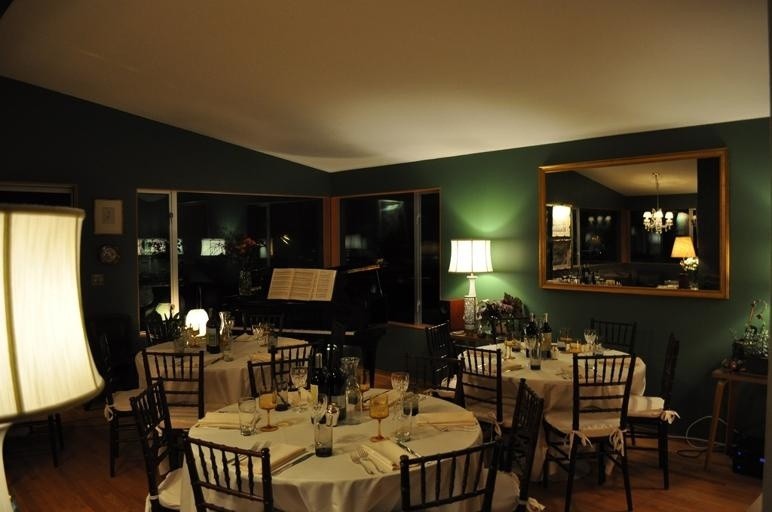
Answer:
[{"left": 726, "top": 296, "right": 770, "bottom": 360}]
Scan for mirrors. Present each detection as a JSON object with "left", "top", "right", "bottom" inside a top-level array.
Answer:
[{"left": 535, "top": 143, "right": 732, "bottom": 302}]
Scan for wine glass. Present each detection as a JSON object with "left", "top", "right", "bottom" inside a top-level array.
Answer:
[
  {"left": 304, "top": 392, "right": 328, "bottom": 446},
  {"left": 368, "top": 392, "right": 391, "bottom": 442},
  {"left": 258, "top": 386, "right": 281, "bottom": 433},
  {"left": 357, "top": 368, "right": 370, "bottom": 410},
  {"left": 289, "top": 366, "right": 308, "bottom": 413},
  {"left": 583, "top": 328, "right": 596, "bottom": 352}
]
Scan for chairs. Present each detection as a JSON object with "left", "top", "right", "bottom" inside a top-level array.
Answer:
[
  {"left": 180, "top": 431, "right": 273, "bottom": 511},
  {"left": 619, "top": 333, "right": 682, "bottom": 491},
  {"left": 7, "top": 410, "right": 68, "bottom": 471},
  {"left": 425, "top": 321, "right": 455, "bottom": 395},
  {"left": 458, "top": 346, "right": 505, "bottom": 424},
  {"left": 128, "top": 373, "right": 189, "bottom": 512},
  {"left": 550, "top": 254, "right": 691, "bottom": 288},
  {"left": 400, "top": 436, "right": 505, "bottom": 512},
  {"left": 91, "top": 330, "right": 149, "bottom": 479},
  {"left": 475, "top": 378, "right": 544, "bottom": 512},
  {"left": 140, "top": 348, "right": 232, "bottom": 472},
  {"left": 544, "top": 349, "right": 637, "bottom": 512},
  {"left": 248, "top": 320, "right": 349, "bottom": 398},
  {"left": 403, "top": 354, "right": 466, "bottom": 409},
  {"left": 589, "top": 319, "right": 640, "bottom": 357}
]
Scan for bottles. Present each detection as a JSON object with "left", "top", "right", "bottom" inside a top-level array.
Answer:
[
  {"left": 309, "top": 340, "right": 346, "bottom": 426},
  {"left": 206, "top": 304, "right": 231, "bottom": 355},
  {"left": 341, "top": 356, "right": 364, "bottom": 426},
  {"left": 550, "top": 342, "right": 560, "bottom": 360},
  {"left": 539, "top": 312, "right": 552, "bottom": 359},
  {"left": 524, "top": 312, "right": 540, "bottom": 358}
]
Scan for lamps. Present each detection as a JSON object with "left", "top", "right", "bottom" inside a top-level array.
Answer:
[
  {"left": 447, "top": 235, "right": 498, "bottom": 333},
  {"left": 668, "top": 233, "right": 697, "bottom": 261},
  {"left": 0, "top": 200, "right": 115, "bottom": 512},
  {"left": 640, "top": 171, "right": 674, "bottom": 237}
]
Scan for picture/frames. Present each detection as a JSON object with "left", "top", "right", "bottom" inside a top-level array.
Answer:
[{"left": 91, "top": 194, "right": 126, "bottom": 238}]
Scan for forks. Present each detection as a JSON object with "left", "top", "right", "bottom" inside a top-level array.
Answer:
[
  {"left": 355, "top": 446, "right": 385, "bottom": 474},
  {"left": 225, "top": 438, "right": 274, "bottom": 465},
  {"left": 350, "top": 448, "right": 375, "bottom": 477},
  {"left": 429, "top": 420, "right": 480, "bottom": 434}
]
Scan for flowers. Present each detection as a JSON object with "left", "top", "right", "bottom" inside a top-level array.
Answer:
[{"left": 229, "top": 230, "right": 260, "bottom": 272}]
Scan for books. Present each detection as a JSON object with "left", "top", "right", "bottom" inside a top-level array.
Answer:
[{"left": 267, "top": 268, "right": 338, "bottom": 300}]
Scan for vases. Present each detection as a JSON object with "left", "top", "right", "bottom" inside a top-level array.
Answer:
[{"left": 241, "top": 268, "right": 253, "bottom": 297}]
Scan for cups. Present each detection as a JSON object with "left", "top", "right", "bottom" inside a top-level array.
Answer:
[
  {"left": 557, "top": 327, "right": 569, "bottom": 351},
  {"left": 495, "top": 336, "right": 507, "bottom": 359},
  {"left": 312, "top": 413, "right": 336, "bottom": 457},
  {"left": 390, "top": 371, "right": 410, "bottom": 395},
  {"left": 236, "top": 396, "right": 257, "bottom": 436},
  {"left": 267, "top": 324, "right": 279, "bottom": 354},
  {"left": 510, "top": 329, "right": 522, "bottom": 351},
  {"left": 401, "top": 394, "right": 420, "bottom": 415},
  {"left": 568, "top": 338, "right": 581, "bottom": 354},
  {"left": 527, "top": 345, "right": 543, "bottom": 371},
  {"left": 252, "top": 321, "right": 267, "bottom": 345},
  {"left": 393, "top": 401, "right": 412, "bottom": 442},
  {"left": 274, "top": 381, "right": 289, "bottom": 411},
  {"left": 173, "top": 338, "right": 200, "bottom": 359}
]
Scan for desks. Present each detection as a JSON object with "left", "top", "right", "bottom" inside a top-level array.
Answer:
[
  {"left": 135, "top": 333, "right": 314, "bottom": 410},
  {"left": 187, "top": 385, "right": 486, "bottom": 512},
  {"left": 701, "top": 365, "right": 769, "bottom": 478},
  {"left": 457, "top": 341, "right": 647, "bottom": 410}
]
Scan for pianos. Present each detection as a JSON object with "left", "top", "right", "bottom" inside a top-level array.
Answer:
[{"left": 215, "top": 264, "right": 387, "bottom": 389}]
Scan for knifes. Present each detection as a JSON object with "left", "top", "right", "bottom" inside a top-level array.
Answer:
[
  {"left": 416, "top": 421, "right": 478, "bottom": 428},
  {"left": 195, "top": 424, "right": 241, "bottom": 430},
  {"left": 387, "top": 394, "right": 414, "bottom": 408},
  {"left": 260, "top": 448, "right": 306, "bottom": 474},
  {"left": 393, "top": 440, "right": 421, "bottom": 458},
  {"left": 272, "top": 451, "right": 317, "bottom": 476}
]
